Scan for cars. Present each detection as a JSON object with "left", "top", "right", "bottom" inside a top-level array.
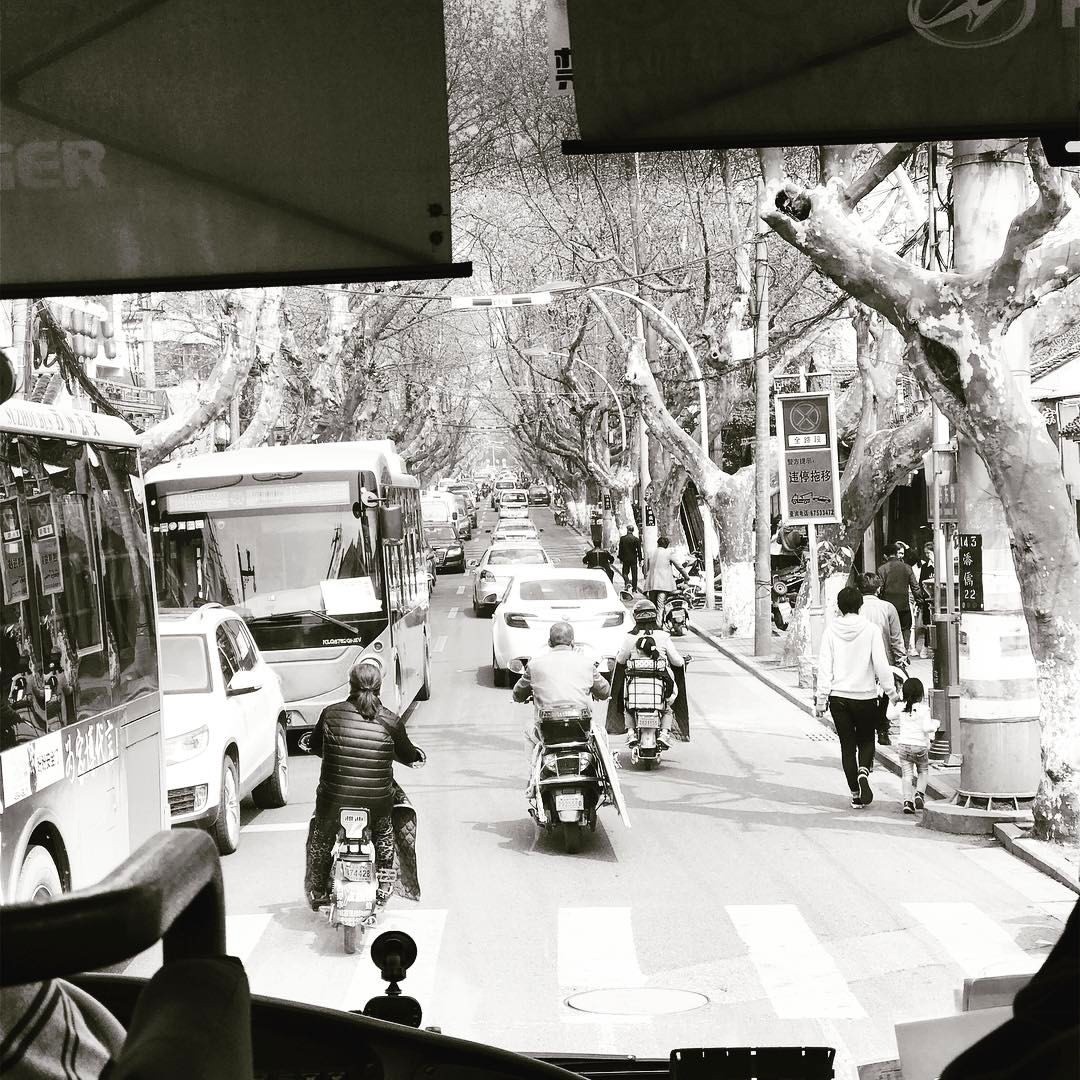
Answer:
[
  {"left": 467, "top": 541, "right": 562, "bottom": 617},
  {"left": 419, "top": 471, "right": 553, "bottom": 576},
  {"left": 481, "top": 566, "right": 636, "bottom": 690}
]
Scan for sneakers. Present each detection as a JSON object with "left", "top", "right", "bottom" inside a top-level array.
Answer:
[{"left": 851, "top": 769, "right": 874, "bottom": 808}]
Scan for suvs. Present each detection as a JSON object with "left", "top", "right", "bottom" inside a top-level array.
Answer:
[{"left": 156, "top": 599, "right": 290, "bottom": 859}]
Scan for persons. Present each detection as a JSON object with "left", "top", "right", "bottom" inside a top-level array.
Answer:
[
  {"left": 857, "top": 572, "right": 909, "bottom": 745},
  {"left": 515, "top": 478, "right": 530, "bottom": 489},
  {"left": 616, "top": 600, "right": 684, "bottom": 667},
  {"left": 777, "top": 527, "right": 803, "bottom": 553},
  {"left": 513, "top": 622, "right": 611, "bottom": 757},
  {"left": 878, "top": 544, "right": 923, "bottom": 655},
  {"left": 624, "top": 637, "right": 679, "bottom": 748},
  {"left": 305, "top": 665, "right": 423, "bottom": 910},
  {"left": 583, "top": 540, "right": 614, "bottom": 583},
  {"left": 895, "top": 542, "right": 934, "bottom": 659},
  {"left": 887, "top": 678, "right": 940, "bottom": 815},
  {"left": 815, "top": 586, "right": 898, "bottom": 809},
  {"left": 617, "top": 526, "right": 643, "bottom": 592},
  {"left": 643, "top": 537, "right": 689, "bottom": 627}
]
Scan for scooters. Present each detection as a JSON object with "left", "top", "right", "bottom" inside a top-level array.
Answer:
[
  {"left": 298, "top": 730, "right": 397, "bottom": 955},
  {"left": 506, "top": 658, "right": 612, "bottom": 855},
  {"left": 660, "top": 541, "right": 810, "bottom": 638},
  {"left": 607, "top": 651, "right": 693, "bottom": 773}
]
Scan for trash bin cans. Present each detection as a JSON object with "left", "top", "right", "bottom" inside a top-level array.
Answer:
[{"left": 590, "top": 524, "right": 602, "bottom": 541}]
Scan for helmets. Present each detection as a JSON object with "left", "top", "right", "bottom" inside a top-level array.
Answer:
[{"left": 632, "top": 599, "right": 658, "bottom": 616}]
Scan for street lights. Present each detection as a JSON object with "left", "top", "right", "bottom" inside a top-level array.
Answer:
[
  {"left": 530, "top": 279, "right": 716, "bottom": 614},
  {"left": 520, "top": 347, "right": 628, "bottom": 470}
]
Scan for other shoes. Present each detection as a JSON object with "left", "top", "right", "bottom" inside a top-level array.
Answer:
[
  {"left": 657, "top": 733, "right": 672, "bottom": 748},
  {"left": 625, "top": 734, "right": 638, "bottom": 744},
  {"left": 903, "top": 792, "right": 926, "bottom": 814}
]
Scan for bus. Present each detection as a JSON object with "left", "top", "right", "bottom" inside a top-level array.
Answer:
[
  {"left": 0, "top": 397, "right": 173, "bottom": 990},
  {"left": 140, "top": 436, "right": 435, "bottom": 759}
]
